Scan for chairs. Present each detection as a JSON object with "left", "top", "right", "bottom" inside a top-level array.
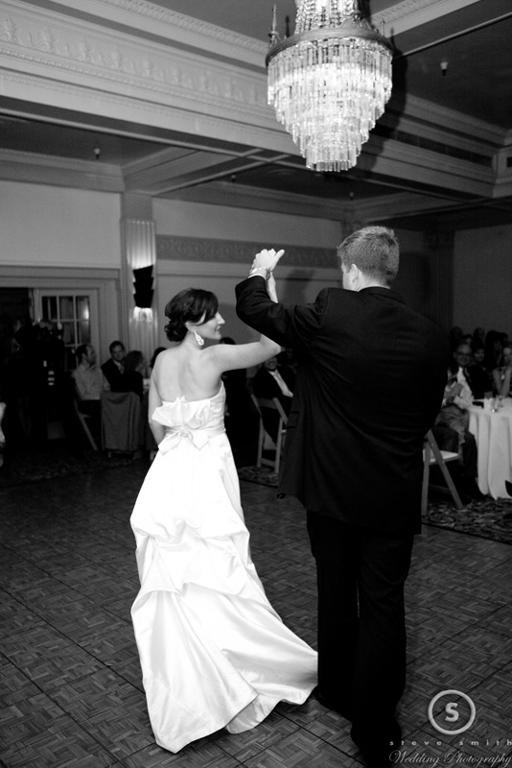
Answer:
[
  {"left": 421, "top": 430, "right": 464, "bottom": 516},
  {"left": 249, "top": 393, "right": 289, "bottom": 475},
  {"left": 65, "top": 379, "right": 159, "bottom": 462}
]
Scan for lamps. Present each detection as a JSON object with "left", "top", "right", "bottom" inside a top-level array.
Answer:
[
  {"left": 265, "top": 1, "right": 394, "bottom": 174},
  {"left": 132, "top": 264, "right": 153, "bottom": 308}
]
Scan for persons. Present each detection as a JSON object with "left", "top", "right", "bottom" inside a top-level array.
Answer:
[
  {"left": 129, "top": 226, "right": 447, "bottom": 767},
  {"left": 0, "top": 315, "right": 144, "bottom": 454},
  {"left": 432, "top": 326, "right": 512, "bottom": 503}
]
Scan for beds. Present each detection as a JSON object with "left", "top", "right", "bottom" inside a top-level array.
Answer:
[{"left": 466, "top": 397, "right": 511, "bottom": 500}]
